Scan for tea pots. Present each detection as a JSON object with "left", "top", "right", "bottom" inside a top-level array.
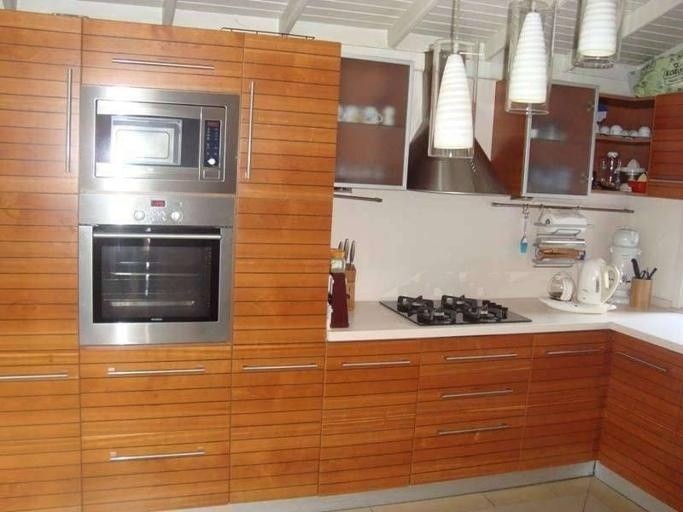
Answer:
[{"left": 547, "top": 270, "right": 576, "bottom": 302}]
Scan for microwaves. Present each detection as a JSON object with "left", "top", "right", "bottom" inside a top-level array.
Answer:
[{"left": 78, "top": 85, "right": 237, "bottom": 193}]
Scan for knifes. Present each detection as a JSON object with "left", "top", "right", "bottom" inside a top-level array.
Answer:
[
  {"left": 630, "top": 258, "right": 642, "bottom": 280},
  {"left": 338, "top": 240, "right": 356, "bottom": 268}
]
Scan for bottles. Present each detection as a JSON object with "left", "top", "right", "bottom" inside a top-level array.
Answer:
[
  {"left": 600, "top": 151, "right": 622, "bottom": 192},
  {"left": 331, "top": 248, "right": 346, "bottom": 274}
]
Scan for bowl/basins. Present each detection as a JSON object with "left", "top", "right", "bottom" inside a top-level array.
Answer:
[{"left": 595, "top": 123, "right": 651, "bottom": 137}]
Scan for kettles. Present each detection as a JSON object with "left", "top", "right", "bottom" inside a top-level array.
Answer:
[{"left": 576, "top": 253, "right": 620, "bottom": 303}]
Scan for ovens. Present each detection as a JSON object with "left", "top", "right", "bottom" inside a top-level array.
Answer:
[{"left": 78, "top": 203, "right": 233, "bottom": 344}]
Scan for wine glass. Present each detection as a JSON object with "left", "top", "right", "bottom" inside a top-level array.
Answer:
[{"left": 622, "top": 163, "right": 644, "bottom": 182}]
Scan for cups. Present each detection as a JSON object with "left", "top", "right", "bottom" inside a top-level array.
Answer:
[{"left": 335, "top": 103, "right": 397, "bottom": 126}]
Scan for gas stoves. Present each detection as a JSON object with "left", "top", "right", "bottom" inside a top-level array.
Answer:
[{"left": 379, "top": 292, "right": 531, "bottom": 326}]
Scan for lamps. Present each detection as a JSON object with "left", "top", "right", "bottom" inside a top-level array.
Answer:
[{"left": 427, "top": 0, "right": 628, "bottom": 160}]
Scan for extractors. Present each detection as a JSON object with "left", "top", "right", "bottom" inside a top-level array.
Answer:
[{"left": 408, "top": 50, "right": 511, "bottom": 200}]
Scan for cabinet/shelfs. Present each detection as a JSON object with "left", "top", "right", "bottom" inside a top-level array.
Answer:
[
  {"left": 2, "top": 9, "right": 342, "bottom": 512},
  {"left": 324, "top": 328, "right": 682, "bottom": 512},
  {"left": 332, "top": 56, "right": 683, "bottom": 203}
]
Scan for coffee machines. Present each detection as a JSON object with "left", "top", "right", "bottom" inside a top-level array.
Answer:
[{"left": 610, "top": 225, "right": 642, "bottom": 281}]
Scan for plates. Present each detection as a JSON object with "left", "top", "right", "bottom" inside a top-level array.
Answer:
[{"left": 538, "top": 296, "right": 616, "bottom": 313}]
